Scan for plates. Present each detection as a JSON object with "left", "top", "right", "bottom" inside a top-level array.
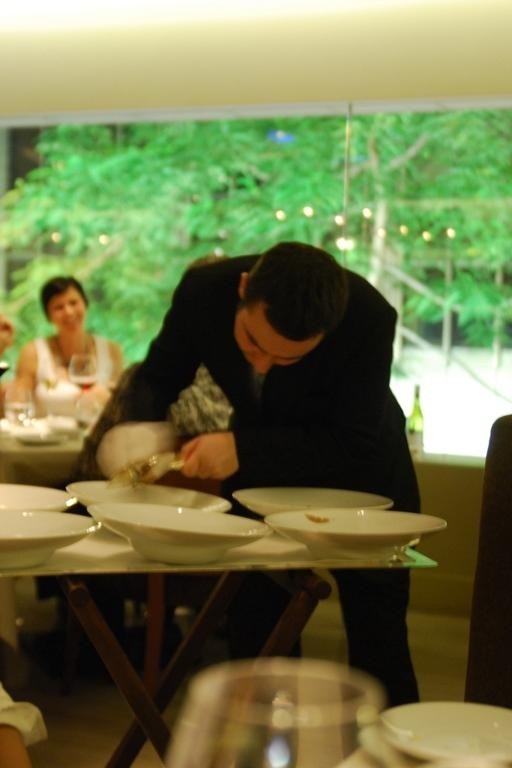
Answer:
[
  {"left": 379, "top": 700, "right": 512, "bottom": 762},
  {"left": 90, "top": 503, "right": 271, "bottom": 565},
  {"left": 1, "top": 483, "right": 77, "bottom": 514},
  {"left": 0, "top": 510, "right": 103, "bottom": 571},
  {"left": 68, "top": 479, "right": 233, "bottom": 514},
  {"left": 265, "top": 509, "right": 449, "bottom": 561},
  {"left": 231, "top": 486, "right": 393, "bottom": 519}
]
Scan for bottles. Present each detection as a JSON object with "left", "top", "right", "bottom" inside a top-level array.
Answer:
[{"left": 406, "top": 385, "right": 424, "bottom": 454}]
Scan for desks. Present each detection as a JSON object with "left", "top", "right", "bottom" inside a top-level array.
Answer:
[{"left": 0, "top": 528, "right": 436, "bottom": 768}]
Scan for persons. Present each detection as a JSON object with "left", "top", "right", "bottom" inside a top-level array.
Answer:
[
  {"left": 14, "top": 275, "right": 123, "bottom": 422},
  {"left": 96, "top": 244, "right": 423, "bottom": 758}
]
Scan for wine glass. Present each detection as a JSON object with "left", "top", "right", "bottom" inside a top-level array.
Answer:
[{"left": 0, "top": 352, "right": 108, "bottom": 447}]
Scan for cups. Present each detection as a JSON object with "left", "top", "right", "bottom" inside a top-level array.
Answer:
[{"left": 165, "top": 657, "right": 389, "bottom": 768}]
